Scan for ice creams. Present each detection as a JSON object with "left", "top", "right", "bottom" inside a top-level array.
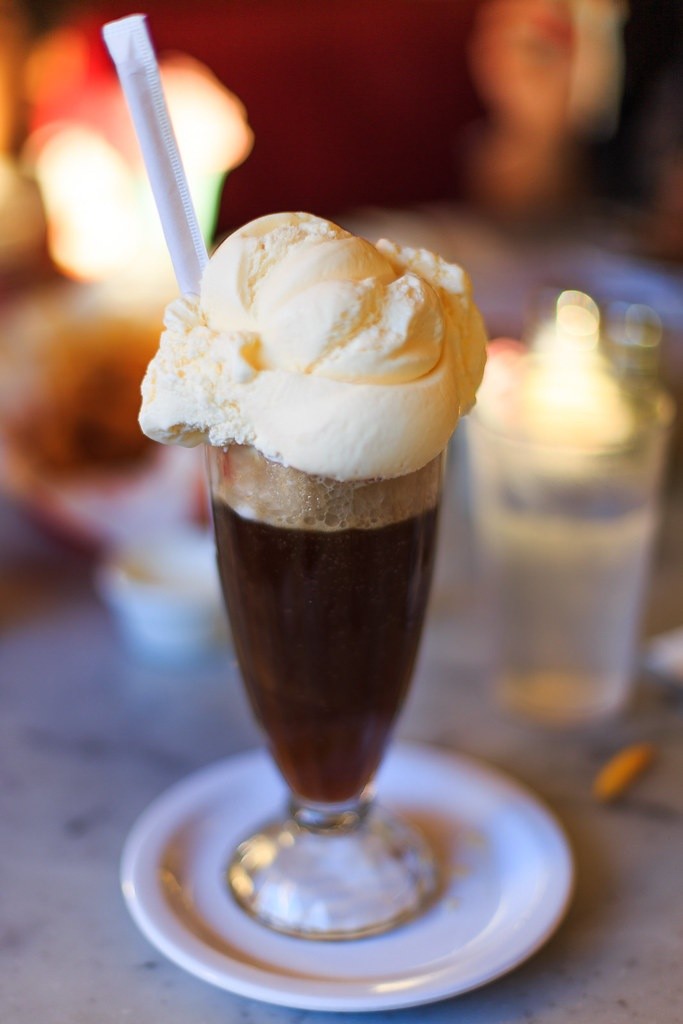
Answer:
[{"left": 135, "top": 210, "right": 487, "bottom": 481}]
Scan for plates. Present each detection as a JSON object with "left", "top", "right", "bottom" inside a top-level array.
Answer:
[{"left": 120, "top": 732, "right": 578, "bottom": 1010}]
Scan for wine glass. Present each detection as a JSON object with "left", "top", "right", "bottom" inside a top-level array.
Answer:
[{"left": 196, "top": 429, "right": 440, "bottom": 945}]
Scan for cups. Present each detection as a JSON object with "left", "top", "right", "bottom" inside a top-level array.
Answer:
[{"left": 466, "top": 371, "right": 673, "bottom": 718}]
